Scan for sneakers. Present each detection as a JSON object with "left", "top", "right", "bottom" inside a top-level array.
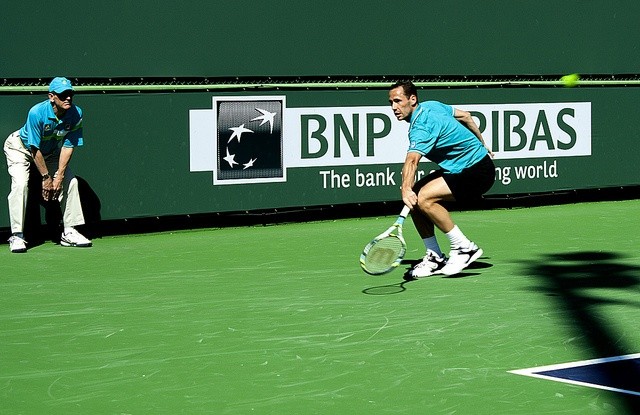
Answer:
[
  {"left": 60, "top": 229, "right": 92, "bottom": 247},
  {"left": 7, "top": 234, "right": 28, "bottom": 252},
  {"left": 441, "top": 241, "right": 483, "bottom": 275},
  {"left": 409, "top": 253, "right": 446, "bottom": 277}
]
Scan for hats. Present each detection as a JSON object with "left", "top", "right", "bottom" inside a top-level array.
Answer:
[{"left": 48, "top": 77, "right": 76, "bottom": 95}]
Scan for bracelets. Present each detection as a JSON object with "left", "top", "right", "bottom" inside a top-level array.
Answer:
[
  {"left": 40, "top": 172, "right": 51, "bottom": 181},
  {"left": 53, "top": 170, "right": 65, "bottom": 178}
]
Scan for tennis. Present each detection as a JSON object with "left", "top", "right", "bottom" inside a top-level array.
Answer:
[{"left": 559, "top": 73, "right": 580, "bottom": 87}]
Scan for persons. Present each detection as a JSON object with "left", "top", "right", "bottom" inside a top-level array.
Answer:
[
  {"left": 388, "top": 80, "right": 495, "bottom": 278},
  {"left": 4, "top": 76, "right": 92, "bottom": 253}
]
